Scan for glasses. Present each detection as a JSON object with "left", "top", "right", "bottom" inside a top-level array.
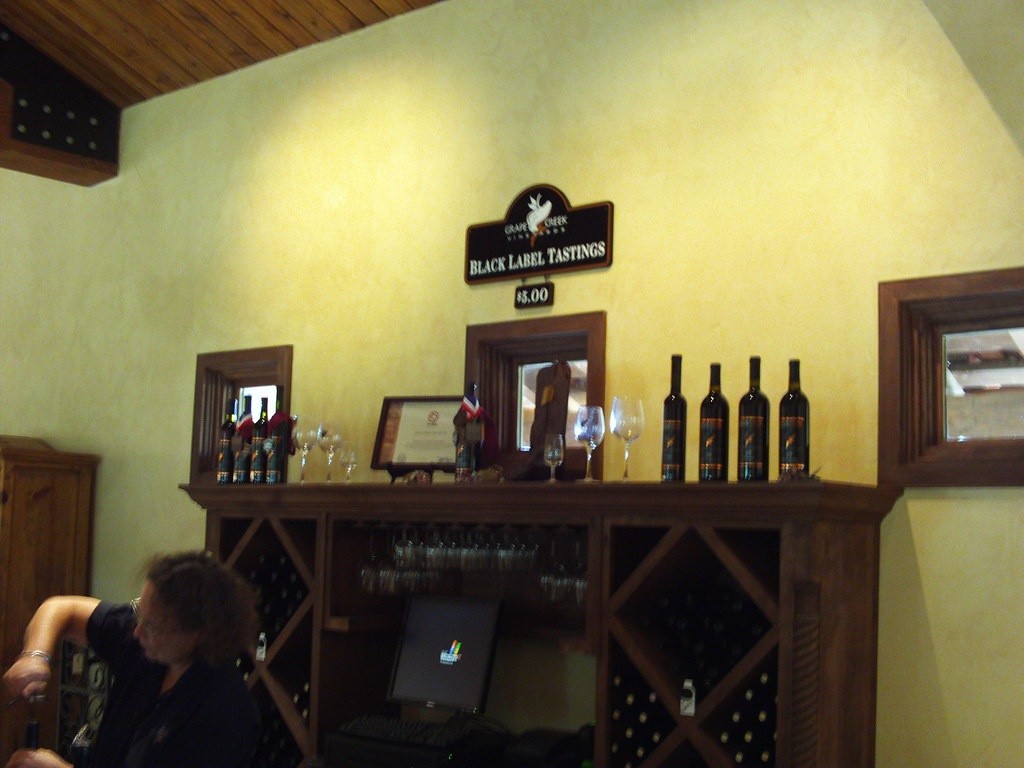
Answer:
[{"left": 129, "top": 596, "right": 166, "bottom": 640}]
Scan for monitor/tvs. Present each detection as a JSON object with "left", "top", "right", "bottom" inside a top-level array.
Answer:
[{"left": 385, "top": 590, "right": 505, "bottom": 716}]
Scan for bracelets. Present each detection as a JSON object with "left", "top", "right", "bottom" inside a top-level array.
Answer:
[{"left": 16, "top": 650, "right": 54, "bottom": 666}]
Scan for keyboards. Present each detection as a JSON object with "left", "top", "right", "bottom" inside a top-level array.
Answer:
[{"left": 339, "top": 713, "right": 471, "bottom": 749}]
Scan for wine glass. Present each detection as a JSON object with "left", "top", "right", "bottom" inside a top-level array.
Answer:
[
  {"left": 543, "top": 434, "right": 564, "bottom": 485},
  {"left": 340, "top": 440, "right": 357, "bottom": 483},
  {"left": 609, "top": 396, "right": 644, "bottom": 482},
  {"left": 317, "top": 421, "right": 342, "bottom": 485},
  {"left": 291, "top": 416, "right": 324, "bottom": 487},
  {"left": 358, "top": 526, "right": 582, "bottom": 606},
  {"left": 574, "top": 405, "right": 606, "bottom": 484}
]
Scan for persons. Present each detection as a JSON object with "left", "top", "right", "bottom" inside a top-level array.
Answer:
[{"left": 0, "top": 552, "right": 267, "bottom": 768}]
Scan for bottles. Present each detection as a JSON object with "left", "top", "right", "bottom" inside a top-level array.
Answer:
[
  {"left": 699, "top": 363, "right": 728, "bottom": 483},
  {"left": 10, "top": 96, "right": 101, "bottom": 158},
  {"left": 23, "top": 719, "right": 38, "bottom": 752},
  {"left": 219, "top": 519, "right": 313, "bottom": 768},
  {"left": 778, "top": 361, "right": 811, "bottom": 482},
  {"left": 660, "top": 355, "right": 687, "bottom": 483},
  {"left": 737, "top": 356, "right": 770, "bottom": 482},
  {"left": 216, "top": 394, "right": 286, "bottom": 485},
  {"left": 609, "top": 527, "right": 776, "bottom": 768}
]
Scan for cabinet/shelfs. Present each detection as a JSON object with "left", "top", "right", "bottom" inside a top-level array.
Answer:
[
  {"left": 0, "top": 449, "right": 101, "bottom": 768},
  {"left": 179, "top": 482, "right": 903, "bottom": 768}
]
